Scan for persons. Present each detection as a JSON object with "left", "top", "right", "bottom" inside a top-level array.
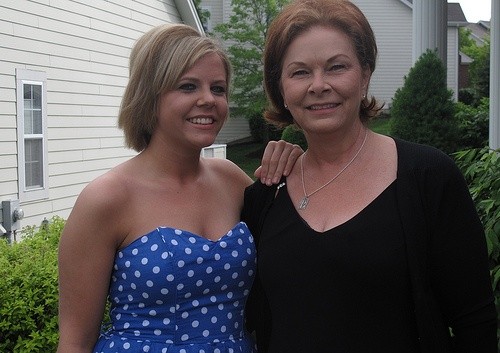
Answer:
[
  {"left": 56, "top": 23, "right": 306, "bottom": 353},
  {"left": 239, "top": 0, "right": 500, "bottom": 353}
]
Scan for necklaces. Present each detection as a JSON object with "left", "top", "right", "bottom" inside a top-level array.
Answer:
[{"left": 297, "top": 126, "right": 369, "bottom": 210}]
